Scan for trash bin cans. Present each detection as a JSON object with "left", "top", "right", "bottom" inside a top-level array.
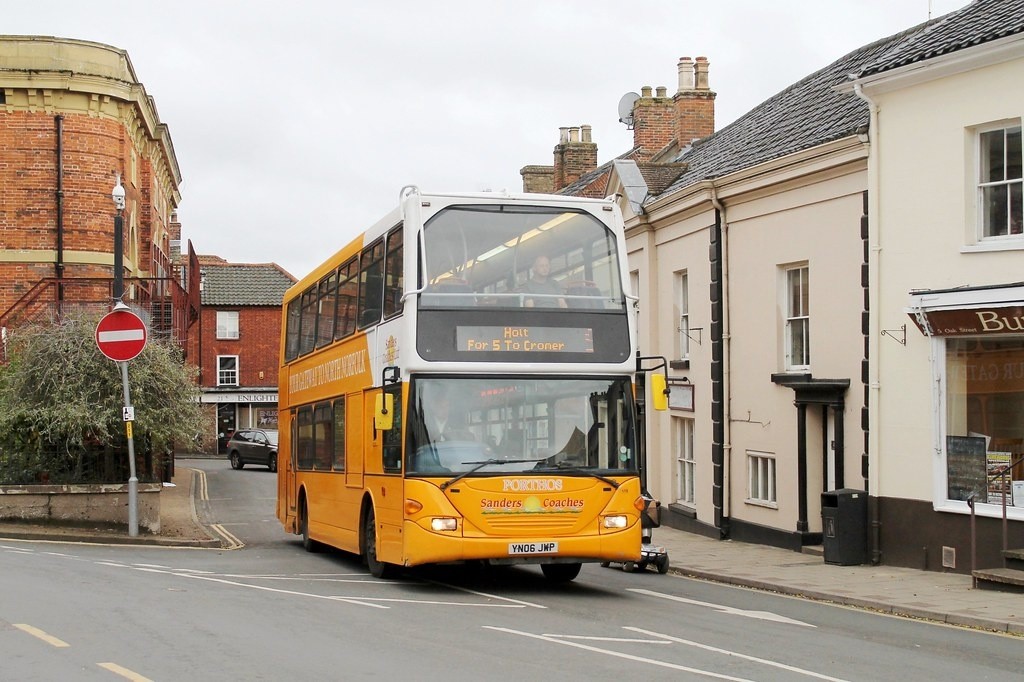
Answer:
[{"left": 819, "top": 488, "right": 867, "bottom": 566}]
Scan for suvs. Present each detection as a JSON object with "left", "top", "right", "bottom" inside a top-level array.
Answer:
[{"left": 226, "top": 427, "right": 280, "bottom": 474}]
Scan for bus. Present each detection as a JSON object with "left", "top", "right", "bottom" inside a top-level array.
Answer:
[{"left": 274, "top": 183, "right": 672, "bottom": 583}]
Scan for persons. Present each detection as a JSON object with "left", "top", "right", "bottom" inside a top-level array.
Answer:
[
  {"left": 520, "top": 254, "right": 568, "bottom": 308},
  {"left": 418, "top": 395, "right": 476, "bottom": 453}
]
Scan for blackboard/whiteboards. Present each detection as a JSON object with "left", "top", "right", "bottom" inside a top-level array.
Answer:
[{"left": 946, "top": 433, "right": 988, "bottom": 505}]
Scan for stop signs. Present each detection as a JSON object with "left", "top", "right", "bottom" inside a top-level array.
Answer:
[{"left": 95, "top": 308, "right": 147, "bottom": 362}]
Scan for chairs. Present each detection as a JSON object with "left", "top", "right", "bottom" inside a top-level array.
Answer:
[{"left": 289, "top": 283, "right": 606, "bottom": 358}]
